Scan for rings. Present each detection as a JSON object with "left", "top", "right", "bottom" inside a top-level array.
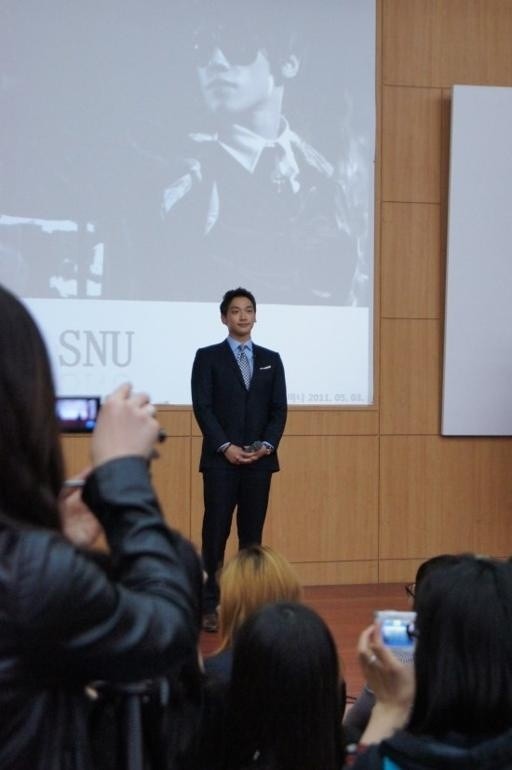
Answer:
[{"left": 363, "top": 653, "right": 382, "bottom": 670}]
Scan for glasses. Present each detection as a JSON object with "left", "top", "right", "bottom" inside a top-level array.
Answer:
[{"left": 192, "top": 38, "right": 261, "bottom": 67}]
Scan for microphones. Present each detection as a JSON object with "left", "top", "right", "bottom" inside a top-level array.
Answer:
[{"left": 244, "top": 441, "right": 262, "bottom": 452}]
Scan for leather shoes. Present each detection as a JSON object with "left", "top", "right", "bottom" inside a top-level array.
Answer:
[{"left": 202, "top": 611, "right": 221, "bottom": 634}]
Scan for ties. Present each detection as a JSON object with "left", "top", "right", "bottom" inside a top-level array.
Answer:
[{"left": 237, "top": 344, "right": 251, "bottom": 392}]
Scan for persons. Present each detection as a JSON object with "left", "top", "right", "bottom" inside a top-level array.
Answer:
[
  {"left": 89, "top": 1, "right": 368, "bottom": 308},
  {"left": 339, "top": 545, "right": 510, "bottom": 770},
  {"left": 0, "top": 285, "right": 199, "bottom": 770},
  {"left": 185, "top": 286, "right": 291, "bottom": 631},
  {"left": 182, "top": 597, "right": 349, "bottom": 770},
  {"left": 188, "top": 542, "right": 298, "bottom": 675},
  {"left": 149, "top": 529, "right": 208, "bottom": 770},
  {"left": 338, "top": 555, "right": 453, "bottom": 746}
]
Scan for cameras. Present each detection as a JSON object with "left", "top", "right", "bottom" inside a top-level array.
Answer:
[
  {"left": 373, "top": 610, "right": 418, "bottom": 663},
  {"left": 55, "top": 397, "right": 100, "bottom": 433}
]
